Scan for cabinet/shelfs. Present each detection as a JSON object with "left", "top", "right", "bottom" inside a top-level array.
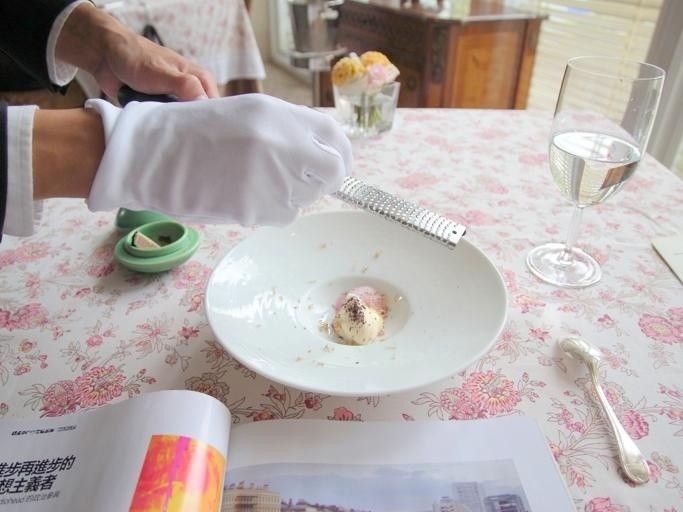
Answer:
[{"left": 332, "top": 1, "right": 547, "bottom": 108}]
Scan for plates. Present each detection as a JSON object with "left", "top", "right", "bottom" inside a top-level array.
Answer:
[{"left": 206, "top": 210, "right": 509, "bottom": 399}]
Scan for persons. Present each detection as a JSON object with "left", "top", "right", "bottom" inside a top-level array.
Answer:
[{"left": 1, "top": 1, "right": 355, "bottom": 242}]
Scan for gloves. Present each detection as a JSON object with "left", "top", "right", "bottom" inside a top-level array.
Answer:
[{"left": 84, "top": 91, "right": 355, "bottom": 226}]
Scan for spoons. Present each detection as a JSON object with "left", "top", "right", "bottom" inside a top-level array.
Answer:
[{"left": 560, "top": 333, "right": 652, "bottom": 491}]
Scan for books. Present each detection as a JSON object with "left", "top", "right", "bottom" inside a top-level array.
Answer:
[{"left": 0, "top": 387, "right": 581, "bottom": 512}]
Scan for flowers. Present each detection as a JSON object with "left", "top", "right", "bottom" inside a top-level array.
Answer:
[{"left": 332, "top": 52, "right": 400, "bottom": 127}]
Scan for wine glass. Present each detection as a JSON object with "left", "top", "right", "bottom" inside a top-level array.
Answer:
[{"left": 527, "top": 55, "right": 666, "bottom": 289}]
